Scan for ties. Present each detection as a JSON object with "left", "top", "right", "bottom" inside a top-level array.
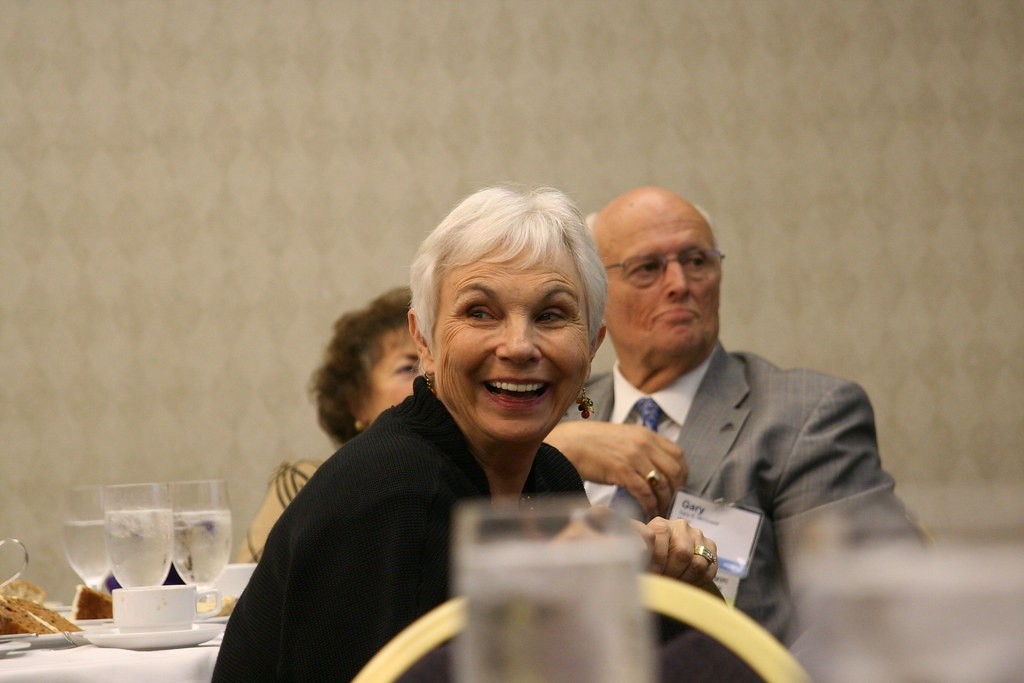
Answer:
[{"left": 606, "top": 397, "right": 664, "bottom": 523}]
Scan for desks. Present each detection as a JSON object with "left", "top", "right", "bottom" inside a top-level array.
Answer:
[{"left": 0, "top": 601, "right": 230, "bottom": 683}]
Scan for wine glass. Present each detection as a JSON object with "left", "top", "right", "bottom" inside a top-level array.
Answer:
[{"left": 60, "top": 484, "right": 112, "bottom": 593}]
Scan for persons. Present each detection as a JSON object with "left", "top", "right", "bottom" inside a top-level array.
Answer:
[
  {"left": 210, "top": 186, "right": 772, "bottom": 683},
  {"left": 548, "top": 187, "right": 943, "bottom": 683},
  {"left": 232, "top": 285, "right": 419, "bottom": 567}
]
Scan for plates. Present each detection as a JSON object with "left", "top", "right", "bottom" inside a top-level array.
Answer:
[
  {"left": 0, "top": 629, "right": 88, "bottom": 649},
  {"left": 0, "top": 641, "right": 32, "bottom": 659},
  {"left": 83, "top": 628, "right": 218, "bottom": 651}
]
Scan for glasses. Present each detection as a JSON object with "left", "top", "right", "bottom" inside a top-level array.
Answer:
[{"left": 605, "top": 250, "right": 726, "bottom": 288}]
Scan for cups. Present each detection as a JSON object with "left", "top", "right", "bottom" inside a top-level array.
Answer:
[
  {"left": 448, "top": 498, "right": 660, "bottom": 683},
  {"left": 111, "top": 584, "right": 222, "bottom": 634},
  {"left": 780, "top": 475, "right": 1024, "bottom": 682},
  {"left": 100, "top": 482, "right": 174, "bottom": 588},
  {"left": 172, "top": 480, "right": 233, "bottom": 584}
]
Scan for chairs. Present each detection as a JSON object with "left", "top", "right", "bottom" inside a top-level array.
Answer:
[{"left": 350, "top": 567, "right": 815, "bottom": 683}]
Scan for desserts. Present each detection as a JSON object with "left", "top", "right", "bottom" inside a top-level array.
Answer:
[
  {"left": 0, "top": 596, "right": 83, "bottom": 635},
  {"left": 71, "top": 585, "right": 114, "bottom": 619}
]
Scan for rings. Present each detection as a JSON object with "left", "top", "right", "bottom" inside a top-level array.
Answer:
[
  {"left": 713, "top": 557, "right": 718, "bottom": 566},
  {"left": 645, "top": 469, "right": 661, "bottom": 487},
  {"left": 694, "top": 545, "right": 714, "bottom": 565}
]
